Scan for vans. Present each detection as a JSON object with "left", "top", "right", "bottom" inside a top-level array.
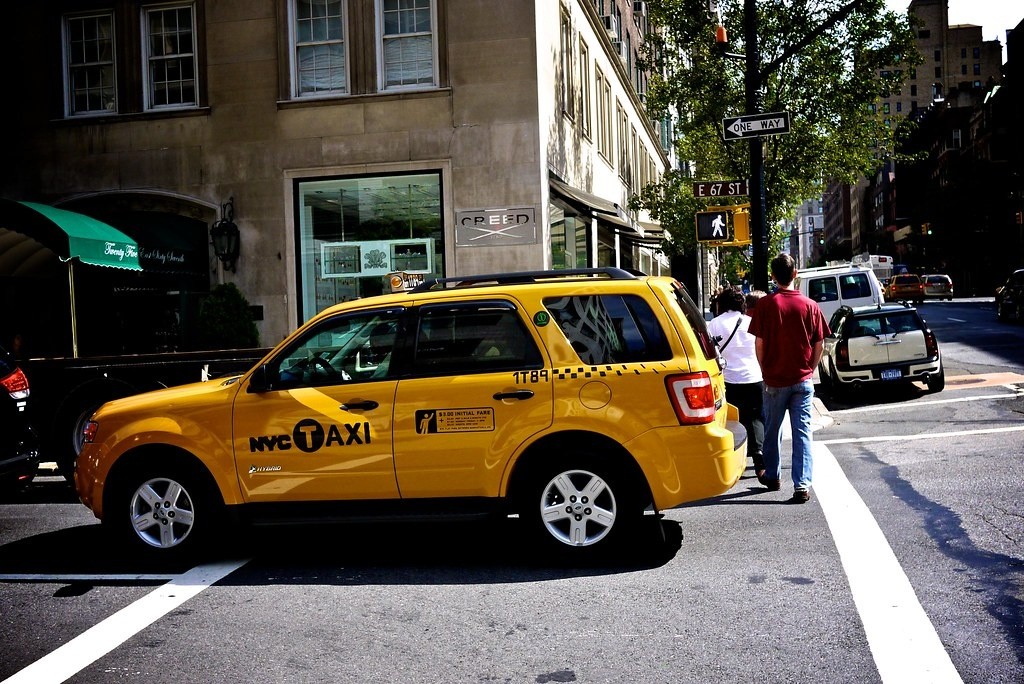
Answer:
[{"left": 797, "top": 266, "right": 885, "bottom": 333}]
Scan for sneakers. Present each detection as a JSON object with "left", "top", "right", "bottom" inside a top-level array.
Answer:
[
  {"left": 753, "top": 450, "right": 763, "bottom": 474},
  {"left": 768, "top": 478, "right": 781, "bottom": 491},
  {"left": 757, "top": 469, "right": 767, "bottom": 483},
  {"left": 792, "top": 489, "right": 809, "bottom": 503}
]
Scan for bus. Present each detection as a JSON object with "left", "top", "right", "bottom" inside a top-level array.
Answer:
[{"left": 852, "top": 255, "right": 894, "bottom": 287}]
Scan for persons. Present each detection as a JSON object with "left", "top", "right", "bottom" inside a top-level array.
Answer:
[
  {"left": 709, "top": 279, "right": 780, "bottom": 316},
  {"left": 746, "top": 252, "right": 831, "bottom": 500},
  {"left": 11, "top": 333, "right": 24, "bottom": 359},
  {"left": 340, "top": 323, "right": 396, "bottom": 381},
  {"left": 704, "top": 289, "right": 767, "bottom": 485}
]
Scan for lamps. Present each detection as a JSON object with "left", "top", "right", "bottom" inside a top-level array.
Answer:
[{"left": 209, "top": 196, "right": 240, "bottom": 274}]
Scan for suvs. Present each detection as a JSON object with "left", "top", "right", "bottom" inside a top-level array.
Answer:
[{"left": 75, "top": 261, "right": 750, "bottom": 580}]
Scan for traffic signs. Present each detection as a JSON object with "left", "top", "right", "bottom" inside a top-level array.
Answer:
[
  {"left": 722, "top": 110, "right": 792, "bottom": 141},
  {"left": 693, "top": 180, "right": 749, "bottom": 199}
]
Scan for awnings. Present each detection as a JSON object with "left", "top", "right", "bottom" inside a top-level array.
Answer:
[
  {"left": 549, "top": 178, "right": 645, "bottom": 238},
  {"left": 635, "top": 221, "right": 672, "bottom": 243}
]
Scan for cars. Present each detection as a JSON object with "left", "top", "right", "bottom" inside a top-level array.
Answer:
[
  {"left": 995, "top": 269, "right": 1024, "bottom": 325},
  {"left": 884, "top": 268, "right": 924, "bottom": 305},
  {"left": 821, "top": 301, "right": 944, "bottom": 401},
  {"left": 920, "top": 274, "right": 953, "bottom": 301},
  {"left": 878, "top": 280, "right": 886, "bottom": 298}
]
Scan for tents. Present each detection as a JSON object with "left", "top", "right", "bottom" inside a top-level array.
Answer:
[{"left": 0, "top": 198, "right": 144, "bottom": 358}]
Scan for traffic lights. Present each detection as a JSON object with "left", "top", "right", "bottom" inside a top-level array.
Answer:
[
  {"left": 819, "top": 233, "right": 824, "bottom": 246},
  {"left": 694, "top": 207, "right": 730, "bottom": 245}
]
